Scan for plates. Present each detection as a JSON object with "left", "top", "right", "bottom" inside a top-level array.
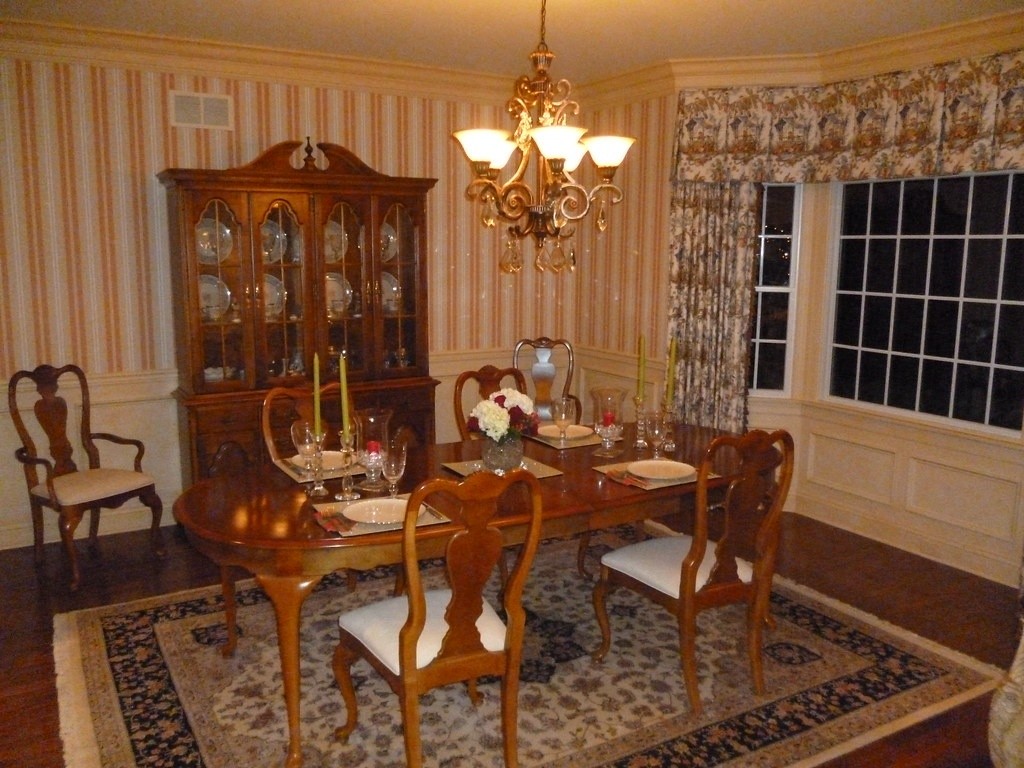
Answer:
[
  {"left": 538, "top": 425, "right": 592, "bottom": 439},
  {"left": 325, "top": 272, "right": 353, "bottom": 314},
  {"left": 380, "top": 222, "right": 397, "bottom": 262},
  {"left": 627, "top": 460, "right": 695, "bottom": 478},
  {"left": 261, "top": 219, "right": 287, "bottom": 265},
  {"left": 263, "top": 273, "right": 286, "bottom": 323},
  {"left": 195, "top": 219, "right": 234, "bottom": 263},
  {"left": 380, "top": 272, "right": 401, "bottom": 310},
  {"left": 343, "top": 499, "right": 426, "bottom": 523},
  {"left": 324, "top": 220, "right": 347, "bottom": 262},
  {"left": 198, "top": 274, "right": 230, "bottom": 319}
]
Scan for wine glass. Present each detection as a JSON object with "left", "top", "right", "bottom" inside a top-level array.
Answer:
[
  {"left": 290, "top": 419, "right": 329, "bottom": 490},
  {"left": 589, "top": 387, "right": 629, "bottom": 456},
  {"left": 378, "top": 438, "right": 407, "bottom": 499},
  {"left": 645, "top": 412, "right": 667, "bottom": 459},
  {"left": 350, "top": 407, "right": 393, "bottom": 489},
  {"left": 551, "top": 398, "right": 576, "bottom": 446}
]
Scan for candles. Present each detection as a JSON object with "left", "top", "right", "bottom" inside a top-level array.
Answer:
[
  {"left": 337, "top": 352, "right": 351, "bottom": 435},
  {"left": 313, "top": 353, "right": 323, "bottom": 435},
  {"left": 637, "top": 335, "right": 646, "bottom": 397},
  {"left": 667, "top": 335, "right": 676, "bottom": 403}
]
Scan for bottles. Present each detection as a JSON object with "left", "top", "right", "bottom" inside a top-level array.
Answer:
[
  {"left": 279, "top": 358, "right": 289, "bottom": 375},
  {"left": 396, "top": 348, "right": 405, "bottom": 366},
  {"left": 328, "top": 345, "right": 337, "bottom": 373},
  {"left": 384, "top": 348, "right": 389, "bottom": 367},
  {"left": 290, "top": 451, "right": 357, "bottom": 470}
]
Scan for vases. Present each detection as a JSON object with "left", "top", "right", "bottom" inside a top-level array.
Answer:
[{"left": 481, "top": 431, "right": 524, "bottom": 469}]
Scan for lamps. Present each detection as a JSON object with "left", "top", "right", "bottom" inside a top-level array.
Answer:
[{"left": 453, "top": 0, "right": 638, "bottom": 269}]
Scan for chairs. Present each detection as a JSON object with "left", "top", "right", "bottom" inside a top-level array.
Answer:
[
  {"left": 454, "top": 365, "right": 593, "bottom": 600},
  {"left": 9, "top": 364, "right": 168, "bottom": 595},
  {"left": 512, "top": 337, "right": 583, "bottom": 429},
  {"left": 332, "top": 468, "right": 541, "bottom": 768},
  {"left": 262, "top": 380, "right": 403, "bottom": 599},
  {"left": 589, "top": 430, "right": 794, "bottom": 714}
]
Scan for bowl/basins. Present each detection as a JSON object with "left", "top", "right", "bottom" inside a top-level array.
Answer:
[{"left": 204, "top": 367, "right": 223, "bottom": 380}]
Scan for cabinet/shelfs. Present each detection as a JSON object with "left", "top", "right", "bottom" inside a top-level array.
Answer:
[{"left": 157, "top": 137, "right": 442, "bottom": 490}]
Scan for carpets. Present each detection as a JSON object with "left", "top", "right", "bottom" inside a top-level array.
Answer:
[{"left": 52, "top": 504, "right": 1009, "bottom": 768}]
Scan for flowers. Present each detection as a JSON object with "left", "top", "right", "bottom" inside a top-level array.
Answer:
[{"left": 466, "top": 386, "right": 539, "bottom": 442}]
